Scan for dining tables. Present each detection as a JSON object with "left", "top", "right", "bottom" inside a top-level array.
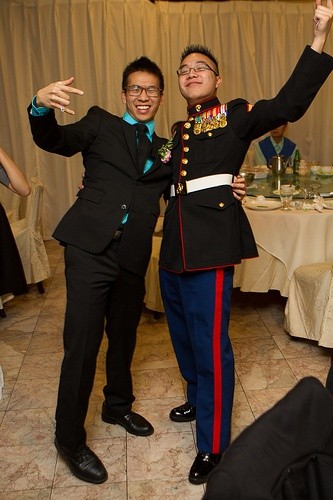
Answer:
[{"left": 235, "top": 161, "right": 333, "bottom": 297}]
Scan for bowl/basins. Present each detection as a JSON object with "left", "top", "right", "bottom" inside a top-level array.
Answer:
[{"left": 239, "top": 172, "right": 256, "bottom": 187}]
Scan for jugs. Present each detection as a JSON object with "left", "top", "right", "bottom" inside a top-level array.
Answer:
[{"left": 267, "top": 153, "right": 290, "bottom": 175}]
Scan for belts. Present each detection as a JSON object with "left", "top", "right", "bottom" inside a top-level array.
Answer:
[
  {"left": 114, "top": 231, "right": 123, "bottom": 240},
  {"left": 170, "top": 173, "right": 237, "bottom": 197}
]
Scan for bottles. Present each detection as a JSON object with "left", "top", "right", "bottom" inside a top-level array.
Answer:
[{"left": 293, "top": 149, "right": 301, "bottom": 174}]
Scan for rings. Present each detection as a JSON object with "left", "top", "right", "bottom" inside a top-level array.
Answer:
[{"left": 61, "top": 107, "right": 64, "bottom": 112}]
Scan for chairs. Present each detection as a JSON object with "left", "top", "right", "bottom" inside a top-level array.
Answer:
[
  {"left": 282, "top": 262, "right": 333, "bottom": 349},
  {"left": 0, "top": 173, "right": 48, "bottom": 317},
  {"left": 143, "top": 218, "right": 166, "bottom": 319}
]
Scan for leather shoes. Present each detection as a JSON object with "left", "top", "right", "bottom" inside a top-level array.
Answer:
[
  {"left": 169, "top": 401, "right": 197, "bottom": 422},
  {"left": 102, "top": 411, "right": 154, "bottom": 436},
  {"left": 189, "top": 449, "right": 223, "bottom": 485},
  {"left": 54, "top": 437, "right": 108, "bottom": 484}
]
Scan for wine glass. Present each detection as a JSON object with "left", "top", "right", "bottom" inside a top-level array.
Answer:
[{"left": 280, "top": 187, "right": 293, "bottom": 211}]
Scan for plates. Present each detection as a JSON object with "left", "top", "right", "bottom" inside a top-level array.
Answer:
[{"left": 246, "top": 201, "right": 285, "bottom": 211}]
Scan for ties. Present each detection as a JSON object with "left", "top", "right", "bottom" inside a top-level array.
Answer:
[
  {"left": 133, "top": 123, "right": 150, "bottom": 178},
  {"left": 275, "top": 145, "right": 280, "bottom": 154}
]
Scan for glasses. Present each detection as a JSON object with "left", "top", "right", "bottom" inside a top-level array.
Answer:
[
  {"left": 123, "top": 85, "right": 163, "bottom": 98},
  {"left": 176, "top": 62, "right": 219, "bottom": 77}
]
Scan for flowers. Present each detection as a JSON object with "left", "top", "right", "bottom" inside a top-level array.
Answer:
[{"left": 157, "top": 133, "right": 176, "bottom": 162}]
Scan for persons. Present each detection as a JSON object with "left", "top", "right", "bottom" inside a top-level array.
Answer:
[
  {"left": 78, "top": 0, "right": 333, "bottom": 483},
  {"left": 254, "top": 122, "right": 296, "bottom": 166},
  {"left": 27, "top": 56, "right": 247, "bottom": 484},
  {"left": 0, "top": 147, "right": 31, "bottom": 296}
]
